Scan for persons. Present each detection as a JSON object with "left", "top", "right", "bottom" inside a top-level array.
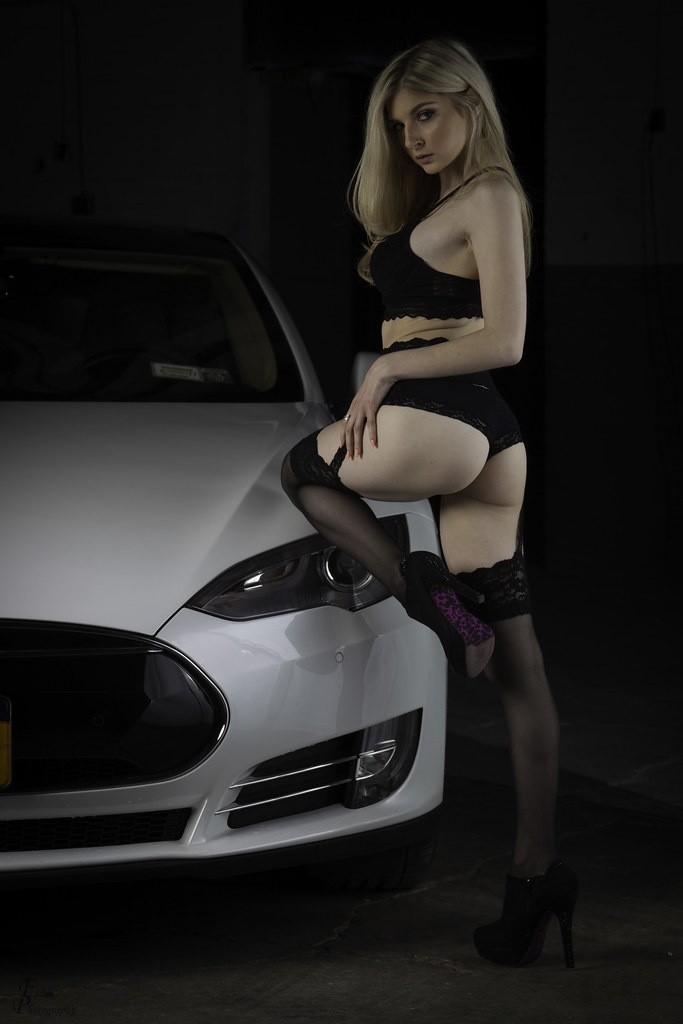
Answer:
[{"left": 282, "top": 39, "right": 577, "bottom": 972}]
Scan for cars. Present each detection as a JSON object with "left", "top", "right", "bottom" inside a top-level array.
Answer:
[{"left": 0, "top": 207, "right": 450, "bottom": 895}]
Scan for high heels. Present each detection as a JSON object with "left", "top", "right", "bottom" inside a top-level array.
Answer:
[
  {"left": 401, "top": 551, "right": 495, "bottom": 682},
  {"left": 472, "top": 851, "right": 581, "bottom": 968}
]
